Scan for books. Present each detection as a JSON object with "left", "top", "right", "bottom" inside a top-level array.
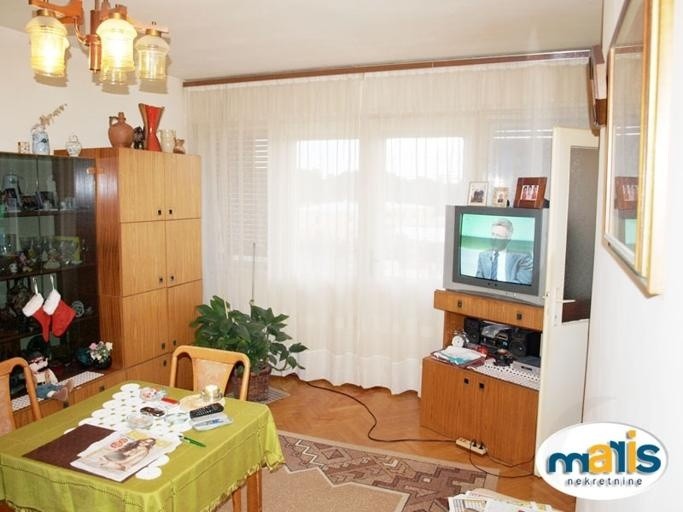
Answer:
[
  {"left": 69, "top": 426, "right": 180, "bottom": 483},
  {"left": 429, "top": 344, "right": 486, "bottom": 371}
]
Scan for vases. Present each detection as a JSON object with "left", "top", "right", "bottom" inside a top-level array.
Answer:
[{"left": 92, "top": 356, "right": 111, "bottom": 370}]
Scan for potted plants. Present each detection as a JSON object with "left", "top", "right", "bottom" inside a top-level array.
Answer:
[{"left": 188, "top": 295, "right": 308, "bottom": 401}]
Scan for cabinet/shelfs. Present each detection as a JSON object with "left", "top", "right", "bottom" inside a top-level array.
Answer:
[
  {"left": 0, "top": 151, "right": 100, "bottom": 400},
  {"left": 13, "top": 399, "right": 54, "bottom": 428},
  {"left": 54, "top": 146, "right": 203, "bottom": 390},
  {"left": 418, "top": 289, "right": 589, "bottom": 475}
]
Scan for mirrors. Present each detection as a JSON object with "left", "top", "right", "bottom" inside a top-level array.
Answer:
[{"left": 600, "top": 0, "right": 673, "bottom": 297}]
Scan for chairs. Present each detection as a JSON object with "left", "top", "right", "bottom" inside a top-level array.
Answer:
[
  {"left": 169, "top": 344, "right": 251, "bottom": 512},
  {"left": 0, "top": 357, "right": 42, "bottom": 436}
]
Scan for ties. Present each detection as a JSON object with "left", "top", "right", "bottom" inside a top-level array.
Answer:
[{"left": 491, "top": 249, "right": 500, "bottom": 280}]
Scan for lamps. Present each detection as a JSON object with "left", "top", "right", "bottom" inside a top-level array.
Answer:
[{"left": 25, "top": 0, "right": 171, "bottom": 86}]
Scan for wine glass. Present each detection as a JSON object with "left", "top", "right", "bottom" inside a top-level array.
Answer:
[{"left": 2, "top": 234, "right": 79, "bottom": 267}]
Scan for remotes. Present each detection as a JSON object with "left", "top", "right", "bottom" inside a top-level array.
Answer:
[{"left": 189, "top": 402, "right": 223, "bottom": 419}]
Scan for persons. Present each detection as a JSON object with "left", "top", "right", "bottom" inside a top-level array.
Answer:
[
  {"left": 497, "top": 193, "right": 505, "bottom": 203},
  {"left": 473, "top": 217, "right": 534, "bottom": 285},
  {"left": 21, "top": 345, "right": 76, "bottom": 402},
  {"left": 521, "top": 184, "right": 538, "bottom": 200}
]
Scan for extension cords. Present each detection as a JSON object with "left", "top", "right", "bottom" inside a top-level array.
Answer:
[{"left": 456, "top": 437, "right": 488, "bottom": 456}]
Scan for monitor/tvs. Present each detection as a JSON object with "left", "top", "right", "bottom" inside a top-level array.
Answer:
[{"left": 442, "top": 204, "right": 548, "bottom": 308}]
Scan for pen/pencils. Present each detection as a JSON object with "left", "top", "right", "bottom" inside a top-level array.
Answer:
[{"left": 178, "top": 435, "right": 205, "bottom": 448}]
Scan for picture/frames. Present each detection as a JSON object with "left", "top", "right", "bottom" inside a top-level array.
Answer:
[
  {"left": 614, "top": 176, "right": 638, "bottom": 211},
  {"left": 465, "top": 175, "right": 548, "bottom": 210}
]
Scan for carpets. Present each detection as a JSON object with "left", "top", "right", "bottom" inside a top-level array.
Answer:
[
  {"left": 225, "top": 384, "right": 290, "bottom": 405},
  {"left": 217, "top": 429, "right": 502, "bottom": 512}
]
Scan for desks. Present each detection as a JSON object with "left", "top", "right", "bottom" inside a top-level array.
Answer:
[{"left": 0, "top": 379, "right": 286, "bottom": 512}]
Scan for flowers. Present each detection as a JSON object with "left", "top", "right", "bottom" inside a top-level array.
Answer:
[{"left": 86, "top": 341, "right": 113, "bottom": 362}]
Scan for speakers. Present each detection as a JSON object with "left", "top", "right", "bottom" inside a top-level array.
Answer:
[
  {"left": 510, "top": 326, "right": 541, "bottom": 357},
  {"left": 463, "top": 317, "right": 480, "bottom": 344}
]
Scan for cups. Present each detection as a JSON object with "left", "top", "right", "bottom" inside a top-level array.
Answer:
[
  {"left": 203, "top": 386, "right": 222, "bottom": 403},
  {"left": 64, "top": 198, "right": 74, "bottom": 209}
]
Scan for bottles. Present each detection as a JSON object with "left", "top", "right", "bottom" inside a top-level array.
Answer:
[{"left": 18, "top": 140, "right": 30, "bottom": 154}]
